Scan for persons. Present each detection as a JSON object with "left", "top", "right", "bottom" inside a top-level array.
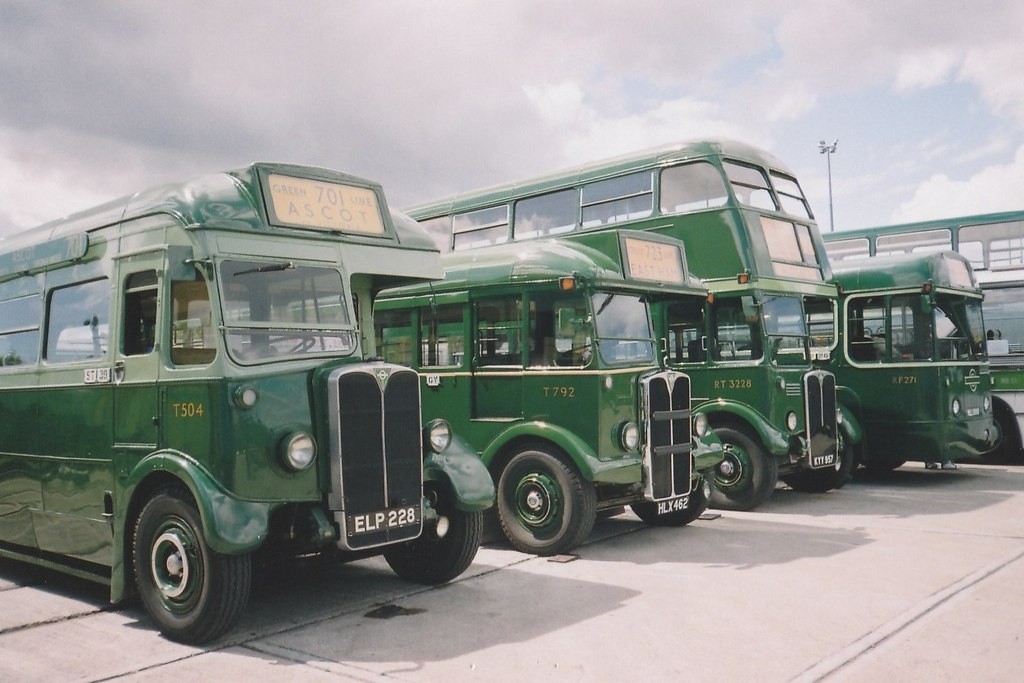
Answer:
[{"left": 925, "top": 460, "right": 957, "bottom": 470}]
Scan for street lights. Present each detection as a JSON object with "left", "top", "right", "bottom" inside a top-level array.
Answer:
[{"left": 819, "top": 138, "right": 840, "bottom": 232}]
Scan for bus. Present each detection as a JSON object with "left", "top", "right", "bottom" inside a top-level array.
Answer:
[{"left": 0, "top": 132, "right": 1024, "bottom": 646}]
